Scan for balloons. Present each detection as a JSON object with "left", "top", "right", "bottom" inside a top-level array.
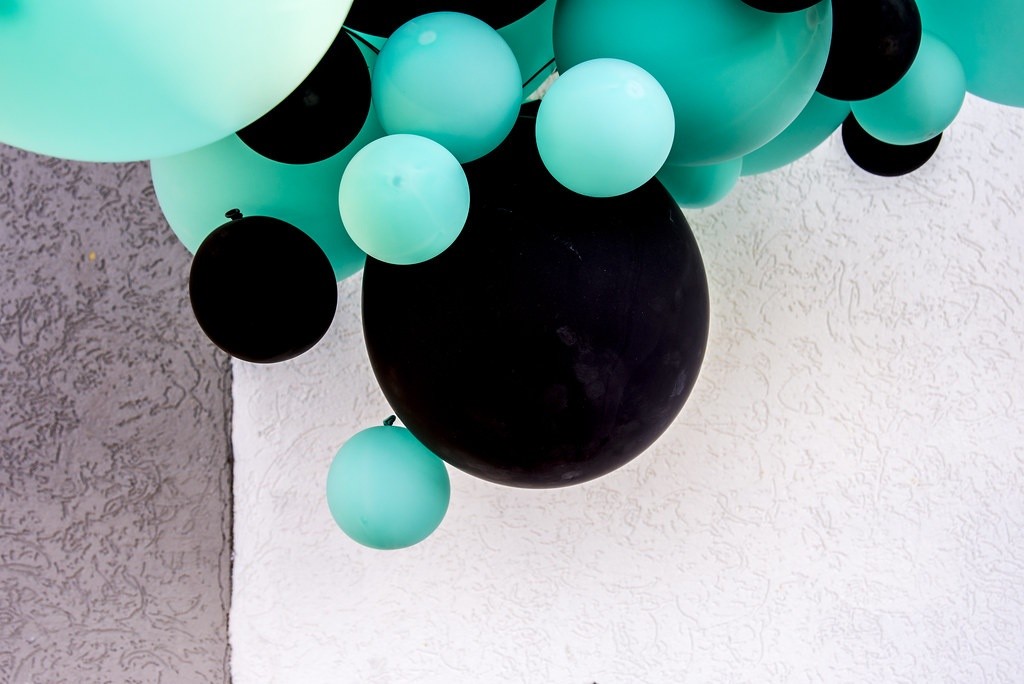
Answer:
[{"left": 1, "top": 1, "right": 1023, "bottom": 553}]
